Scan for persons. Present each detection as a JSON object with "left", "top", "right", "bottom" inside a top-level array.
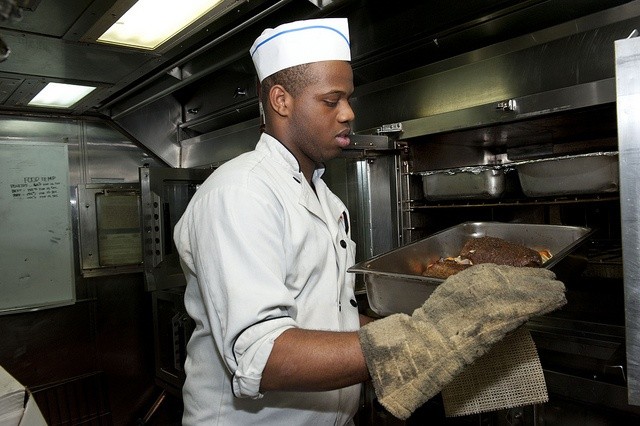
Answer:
[{"left": 173, "top": 18, "right": 588, "bottom": 425}]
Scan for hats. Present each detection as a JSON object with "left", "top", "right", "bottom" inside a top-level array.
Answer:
[{"left": 249, "top": 18, "right": 351, "bottom": 83}]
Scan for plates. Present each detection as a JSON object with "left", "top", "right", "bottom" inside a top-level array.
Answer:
[
  {"left": 418, "top": 163, "right": 518, "bottom": 200},
  {"left": 503, "top": 150, "right": 619, "bottom": 195},
  {"left": 346, "top": 221, "right": 592, "bottom": 317}
]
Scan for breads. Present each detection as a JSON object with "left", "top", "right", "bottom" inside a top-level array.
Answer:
[
  {"left": 419, "top": 256, "right": 475, "bottom": 277},
  {"left": 459, "top": 238, "right": 543, "bottom": 268}
]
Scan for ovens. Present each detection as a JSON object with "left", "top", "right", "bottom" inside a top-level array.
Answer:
[
  {"left": 353, "top": 80, "right": 636, "bottom": 426},
  {"left": 75, "top": 182, "right": 146, "bottom": 278},
  {"left": 139, "top": 136, "right": 392, "bottom": 295}
]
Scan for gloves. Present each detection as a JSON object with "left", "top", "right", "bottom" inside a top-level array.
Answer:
[{"left": 358, "top": 264, "right": 567, "bottom": 415}]
